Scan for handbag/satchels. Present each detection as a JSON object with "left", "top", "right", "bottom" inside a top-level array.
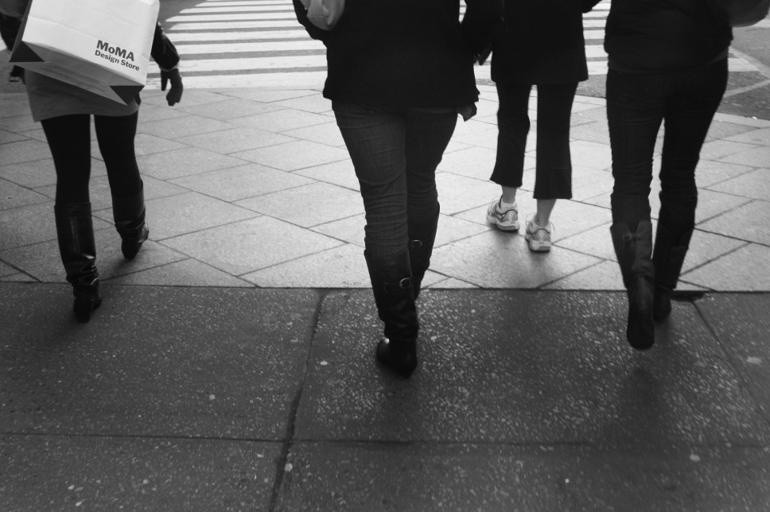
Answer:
[{"left": 301, "top": 0, "right": 345, "bottom": 31}]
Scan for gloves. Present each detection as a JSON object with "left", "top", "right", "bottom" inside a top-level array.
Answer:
[{"left": 160, "top": 68, "right": 184, "bottom": 108}]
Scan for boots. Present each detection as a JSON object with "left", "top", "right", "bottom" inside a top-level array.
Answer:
[
  {"left": 609, "top": 213, "right": 696, "bottom": 349},
  {"left": 53, "top": 205, "right": 104, "bottom": 326},
  {"left": 110, "top": 191, "right": 150, "bottom": 261}
]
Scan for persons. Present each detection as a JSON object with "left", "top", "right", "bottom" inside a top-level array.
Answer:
[
  {"left": 473, "top": 0, "right": 602, "bottom": 253},
  {"left": 0, "top": 0, "right": 182, "bottom": 317},
  {"left": 292, "top": 0, "right": 491, "bottom": 380},
  {"left": 604, "top": 0, "right": 769, "bottom": 351}
]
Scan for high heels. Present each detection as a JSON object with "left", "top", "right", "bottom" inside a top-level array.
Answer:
[{"left": 364, "top": 203, "right": 439, "bottom": 378}]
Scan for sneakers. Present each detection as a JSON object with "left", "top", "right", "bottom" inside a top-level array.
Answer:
[
  {"left": 525, "top": 222, "right": 551, "bottom": 252},
  {"left": 487, "top": 203, "right": 520, "bottom": 232}
]
